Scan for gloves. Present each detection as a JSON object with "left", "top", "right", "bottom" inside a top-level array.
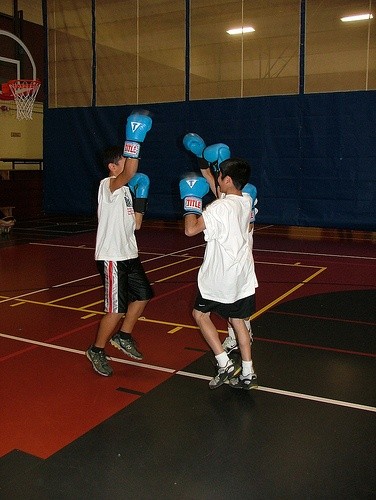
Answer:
[
  {"left": 124, "top": 114, "right": 153, "bottom": 159},
  {"left": 178, "top": 176, "right": 210, "bottom": 217},
  {"left": 128, "top": 173, "right": 150, "bottom": 213},
  {"left": 203, "top": 143, "right": 231, "bottom": 172},
  {"left": 243, "top": 183, "right": 258, "bottom": 223},
  {"left": 182, "top": 133, "right": 210, "bottom": 169}
]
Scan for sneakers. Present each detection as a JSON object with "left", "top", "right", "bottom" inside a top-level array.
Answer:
[
  {"left": 248, "top": 328, "right": 253, "bottom": 346},
  {"left": 86, "top": 345, "right": 112, "bottom": 376},
  {"left": 229, "top": 373, "right": 258, "bottom": 389},
  {"left": 221, "top": 336, "right": 239, "bottom": 355},
  {"left": 109, "top": 334, "right": 143, "bottom": 360},
  {"left": 208, "top": 359, "right": 242, "bottom": 389}
]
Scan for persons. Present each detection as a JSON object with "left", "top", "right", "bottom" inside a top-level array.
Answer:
[
  {"left": 86, "top": 114, "right": 153, "bottom": 376},
  {"left": 179, "top": 132, "right": 258, "bottom": 390}
]
